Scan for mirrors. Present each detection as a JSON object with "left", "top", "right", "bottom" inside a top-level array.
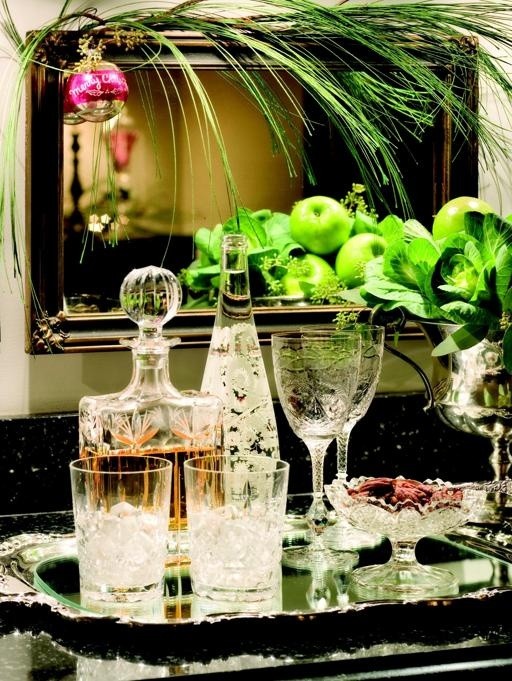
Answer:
[{"left": 24, "top": 31, "right": 480, "bottom": 355}]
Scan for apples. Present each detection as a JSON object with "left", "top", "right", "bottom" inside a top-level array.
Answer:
[
  {"left": 263, "top": 194, "right": 388, "bottom": 294},
  {"left": 432, "top": 195, "right": 500, "bottom": 240}
]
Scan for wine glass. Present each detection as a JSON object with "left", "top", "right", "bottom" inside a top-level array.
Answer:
[
  {"left": 272, "top": 321, "right": 384, "bottom": 556},
  {"left": 322, "top": 478, "right": 483, "bottom": 592}
]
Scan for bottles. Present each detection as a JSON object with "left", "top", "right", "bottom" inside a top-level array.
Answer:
[
  {"left": 78, "top": 265, "right": 226, "bottom": 535},
  {"left": 195, "top": 232, "right": 281, "bottom": 513}
]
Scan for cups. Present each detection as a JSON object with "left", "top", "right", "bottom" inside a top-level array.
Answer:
[
  {"left": 183, "top": 454, "right": 289, "bottom": 603},
  {"left": 69, "top": 456, "right": 171, "bottom": 601}
]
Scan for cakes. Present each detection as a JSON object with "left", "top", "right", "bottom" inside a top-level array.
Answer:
[{"left": 348, "top": 477, "right": 464, "bottom": 506}]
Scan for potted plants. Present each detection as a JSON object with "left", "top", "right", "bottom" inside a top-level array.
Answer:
[{"left": 0, "top": 1, "right": 512, "bottom": 361}]
